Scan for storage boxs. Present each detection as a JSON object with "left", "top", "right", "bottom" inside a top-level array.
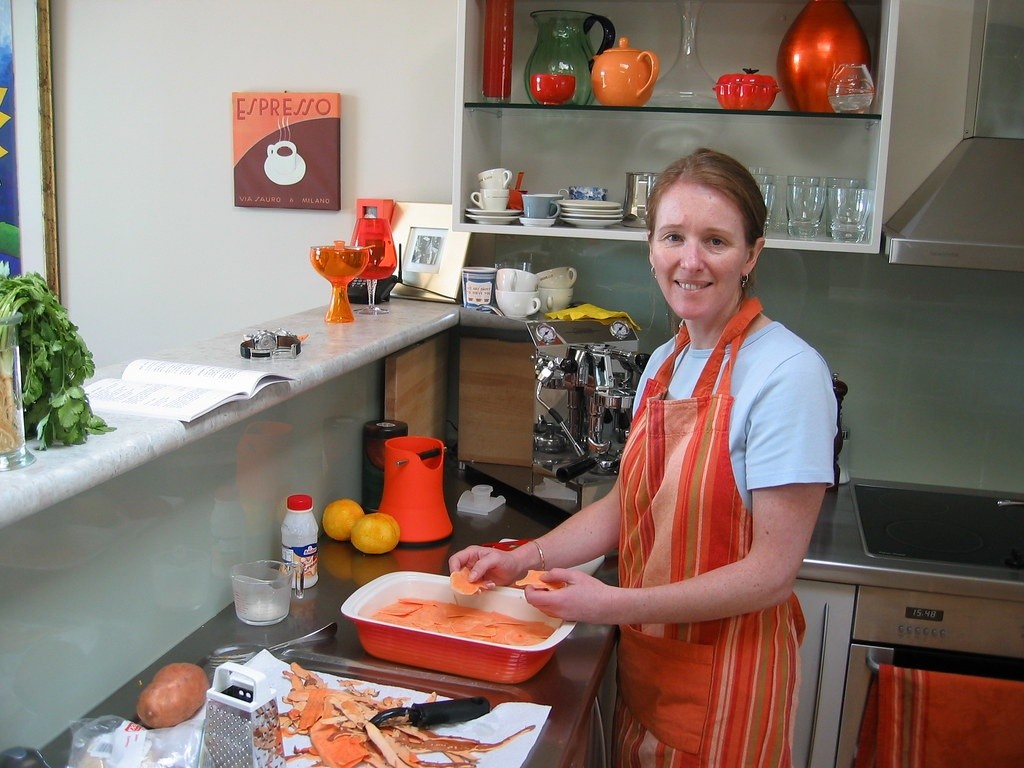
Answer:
[{"left": 342, "top": 568, "right": 578, "bottom": 685}]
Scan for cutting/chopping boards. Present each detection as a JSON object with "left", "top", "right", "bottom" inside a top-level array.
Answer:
[
  {"left": 385, "top": 340, "right": 450, "bottom": 444},
  {"left": 459, "top": 339, "right": 536, "bottom": 464}
]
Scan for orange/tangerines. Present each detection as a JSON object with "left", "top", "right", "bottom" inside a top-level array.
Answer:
[
  {"left": 323, "top": 498, "right": 364, "bottom": 540},
  {"left": 351, "top": 513, "right": 401, "bottom": 554}
]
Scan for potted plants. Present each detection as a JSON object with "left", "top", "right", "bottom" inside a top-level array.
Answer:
[{"left": 0, "top": 275, "right": 114, "bottom": 473}]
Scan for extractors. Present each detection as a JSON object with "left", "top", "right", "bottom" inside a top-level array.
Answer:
[{"left": 879, "top": 0, "right": 1024, "bottom": 273}]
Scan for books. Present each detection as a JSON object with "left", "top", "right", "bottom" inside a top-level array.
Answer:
[{"left": 81, "top": 358, "right": 298, "bottom": 425}]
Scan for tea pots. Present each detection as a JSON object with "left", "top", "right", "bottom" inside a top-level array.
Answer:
[{"left": 590, "top": 38, "right": 659, "bottom": 106}]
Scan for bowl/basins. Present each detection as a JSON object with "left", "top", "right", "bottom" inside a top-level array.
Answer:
[
  {"left": 341, "top": 570, "right": 577, "bottom": 683},
  {"left": 713, "top": 66, "right": 781, "bottom": 110}
]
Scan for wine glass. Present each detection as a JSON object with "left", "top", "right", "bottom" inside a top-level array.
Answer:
[
  {"left": 349, "top": 220, "right": 397, "bottom": 314},
  {"left": 310, "top": 245, "right": 369, "bottom": 323}
]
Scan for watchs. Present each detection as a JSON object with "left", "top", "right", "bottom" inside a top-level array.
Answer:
[{"left": 240, "top": 327, "right": 301, "bottom": 360}]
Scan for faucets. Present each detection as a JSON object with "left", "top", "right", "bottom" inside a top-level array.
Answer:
[{"left": 995, "top": 500, "right": 1024, "bottom": 508}]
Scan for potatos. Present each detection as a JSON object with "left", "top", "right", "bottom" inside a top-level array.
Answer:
[
  {"left": 136, "top": 663, "right": 210, "bottom": 729},
  {"left": 374, "top": 567, "right": 566, "bottom": 647}
]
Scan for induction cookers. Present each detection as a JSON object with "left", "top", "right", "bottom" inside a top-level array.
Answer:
[{"left": 850, "top": 478, "right": 1024, "bottom": 570}]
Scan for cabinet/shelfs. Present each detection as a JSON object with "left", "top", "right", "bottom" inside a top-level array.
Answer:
[
  {"left": 774, "top": 585, "right": 855, "bottom": 768},
  {"left": 448, "top": 0, "right": 914, "bottom": 258}
]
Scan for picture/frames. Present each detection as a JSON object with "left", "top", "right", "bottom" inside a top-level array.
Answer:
[
  {"left": 390, "top": 201, "right": 474, "bottom": 305},
  {"left": 0, "top": 0, "right": 61, "bottom": 305}
]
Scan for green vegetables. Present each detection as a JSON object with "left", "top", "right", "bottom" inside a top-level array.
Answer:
[{"left": 0, "top": 259, "right": 118, "bottom": 450}]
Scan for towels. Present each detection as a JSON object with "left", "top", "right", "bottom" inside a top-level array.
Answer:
[{"left": 852, "top": 661, "right": 1024, "bottom": 768}]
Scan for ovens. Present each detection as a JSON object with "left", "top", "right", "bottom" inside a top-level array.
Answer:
[{"left": 835, "top": 637, "right": 1024, "bottom": 768}]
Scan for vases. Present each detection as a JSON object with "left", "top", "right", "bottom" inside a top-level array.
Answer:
[
  {"left": 776, "top": 0, "right": 873, "bottom": 112},
  {"left": 650, "top": 0, "right": 727, "bottom": 107}
]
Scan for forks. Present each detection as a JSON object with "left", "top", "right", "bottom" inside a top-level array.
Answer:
[{"left": 208, "top": 619, "right": 336, "bottom": 669}]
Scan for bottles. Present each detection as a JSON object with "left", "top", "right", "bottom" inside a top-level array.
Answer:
[
  {"left": 280, "top": 495, "right": 318, "bottom": 588},
  {"left": 646, "top": 0, "right": 723, "bottom": 108},
  {"left": 827, "top": 65, "right": 875, "bottom": 114}
]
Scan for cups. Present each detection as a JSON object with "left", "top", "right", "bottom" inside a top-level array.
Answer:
[
  {"left": 478, "top": 168, "right": 512, "bottom": 190},
  {"left": 557, "top": 185, "right": 608, "bottom": 200},
  {"left": 750, "top": 165, "right": 874, "bottom": 244},
  {"left": 622, "top": 170, "right": 659, "bottom": 228},
  {"left": 470, "top": 188, "right": 510, "bottom": 210},
  {"left": 522, "top": 193, "right": 563, "bottom": 218},
  {"left": 232, "top": 561, "right": 292, "bottom": 625},
  {"left": 461, "top": 258, "right": 576, "bottom": 320}
]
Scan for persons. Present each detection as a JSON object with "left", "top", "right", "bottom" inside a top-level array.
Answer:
[{"left": 449, "top": 148, "right": 838, "bottom": 768}]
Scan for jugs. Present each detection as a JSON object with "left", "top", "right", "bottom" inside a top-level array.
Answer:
[{"left": 523, "top": 9, "right": 615, "bottom": 105}]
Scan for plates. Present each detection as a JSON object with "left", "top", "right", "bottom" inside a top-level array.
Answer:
[{"left": 465, "top": 200, "right": 623, "bottom": 229}]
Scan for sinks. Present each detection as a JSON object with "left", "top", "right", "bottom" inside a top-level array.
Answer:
[{"left": 852, "top": 481, "right": 1024, "bottom": 573}]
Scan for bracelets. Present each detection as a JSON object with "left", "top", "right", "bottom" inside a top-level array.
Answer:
[{"left": 534, "top": 541, "right": 545, "bottom": 571}]
